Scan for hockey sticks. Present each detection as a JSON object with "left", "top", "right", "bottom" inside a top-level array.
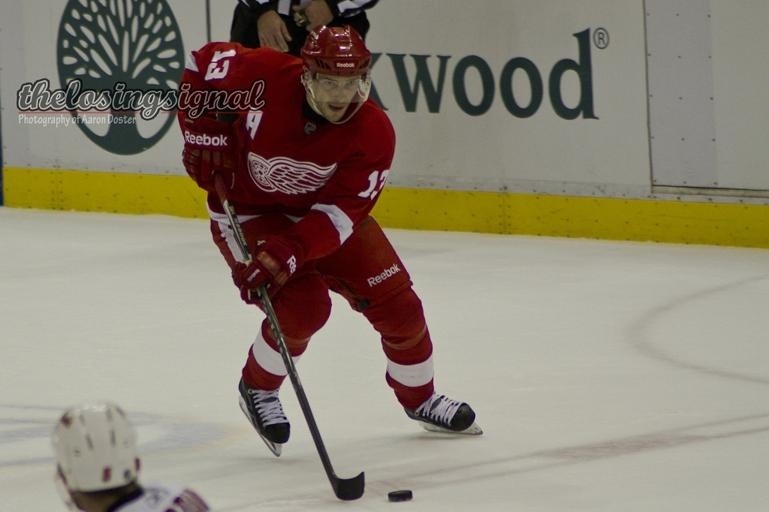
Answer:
[{"left": 214, "top": 174, "right": 365, "bottom": 501}]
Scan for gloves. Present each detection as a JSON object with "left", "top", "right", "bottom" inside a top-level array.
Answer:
[
  {"left": 232, "top": 235, "right": 300, "bottom": 304},
  {"left": 182, "top": 116, "right": 235, "bottom": 191}
]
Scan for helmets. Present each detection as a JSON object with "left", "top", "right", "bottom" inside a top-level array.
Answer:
[
  {"left": 43, "top": 401, "right": 140, "bottom": 512},
  {"left": 301, "top": 25, "right": 372, "bottom": 124}
]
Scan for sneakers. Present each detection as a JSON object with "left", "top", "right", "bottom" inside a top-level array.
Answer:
[
  {"left": 238, "top": 377, "right": 289, "bottom": 443},
  {"left": 404, "top": 393, "right": 475, "bottom": 431}
]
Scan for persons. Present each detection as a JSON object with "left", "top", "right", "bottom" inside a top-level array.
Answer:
[
  {"left": 228, "top": 1, "right": 379, "bottom": 60},
  {"left": 177, "top": 23, "right": 478, "bottom": 444},
  {"left": 48, "top": 401, "right": 209, "bottom": 512}
]
